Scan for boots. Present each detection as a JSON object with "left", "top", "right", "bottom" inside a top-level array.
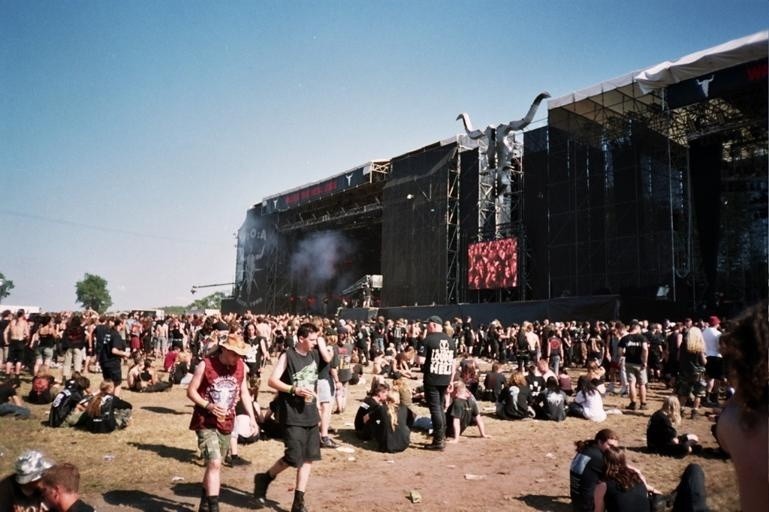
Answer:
[
  {"left": 206, "top": 495, "right": 220, "bottom": 512},
  {"left": 290, "top": 489, "right": 308, "bottom": 512},
  {"left": 198, "top": 486, "right": 209, "bottom": 512},
  {"left": 252, "top": 468, "right": 276, "bottom": 507}
]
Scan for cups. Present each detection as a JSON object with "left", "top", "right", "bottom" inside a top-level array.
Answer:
[
  {"left": 304, "top": 385, "right": 315, "bottom": 404},
  {"left": 216, "top": 402, "right": 229, "bottom": 422},
  {"left": 409, "top": 490, "right": 421, "bottom": 503}
]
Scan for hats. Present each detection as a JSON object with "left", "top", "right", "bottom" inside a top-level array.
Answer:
[
  {"left": 420, "top": 314, "right": 444, "bottom": 326},
  {"left": 707, "top": 315, "right": 720, "bottom": 326},
  {"left": 217, "top": 332, "right": 253, "bottom": 357},
  {"left": 13, "top": 449, "right": 54, "bottom": 486}
]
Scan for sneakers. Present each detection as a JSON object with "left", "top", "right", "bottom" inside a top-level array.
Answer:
[
  {"left": 678, "top": 392, "right": 721, "bottom": 421},
  {"left": 624, "top": 401, "right": 637, "bottom": 411},
  {"left": 319, "top": 435, "right": 343, "bottom": 448},
  {"left": 228, "top": 454, "right": 253, "bottom": 467},
  {"left": 639, "top": 402, "right": 649, "bottom": 410},
  {"left": 422, "top": 439, "right": 445, "bottom": 450}
]
